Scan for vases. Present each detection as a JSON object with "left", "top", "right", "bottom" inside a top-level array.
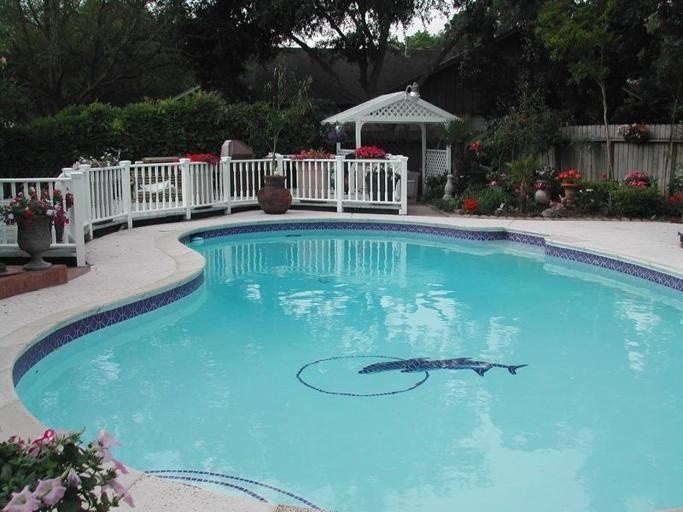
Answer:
[
  {"left": 624, "top": 135, "right": 647, "bottom": 143},
  {"left": 561, "top": 182, "right": 581, "bottom": 205},
  {"left": 359, "top": 161, "right": 382, "bottom": 193},
  {"left": 190, "top": 166, "right": 214, "bottom": 209},
  {"left": 534, "top": 180, "right": 551, "bottom": 204},
  {"left": 295, "top": 163, "right": 328, "bottom": 203},
  {"left": 54, "top": 224, "right": 64, "bottom": 243},
  {"left": 17, "top": 215, "right": 52, "bottom": 271}
]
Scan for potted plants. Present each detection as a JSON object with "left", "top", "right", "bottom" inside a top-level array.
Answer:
[
  {"left": 90, "top": 153, "right": 120, "bottom": 224},
  {"left": 247, "top": 66, "right": 313, "bottom": 214},
  {"left": 362, "top": 159, "right": 400, "bottom": 201}
]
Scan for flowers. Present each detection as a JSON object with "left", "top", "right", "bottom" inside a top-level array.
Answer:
[
  {"left": 0, "top": 185, "right": 69, "bottom": 227},
  {"left": 289, "top": 149, "right": 331, "bottom": 163},
  {"left": 532, "top": 167, "right": 559, "bottom": 188},
  {"left": 617, "top": 123, "right": 650, "bottom": 142},
  {"left": 45, "top": 190, "right": 73, "bottom": 226},
  {"left": 355, "top": 146, "right": 386, "bottom": 165},
  {"left": 177, "top": 153, "right": 221, "bottom": 165},
  {"left": 560, "top": 169, "right": 582, "bottom": 183}
]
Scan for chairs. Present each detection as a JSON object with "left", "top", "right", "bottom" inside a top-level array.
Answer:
[{"left": 407, "top": 171, "right": 420, "bottom": 204}]
